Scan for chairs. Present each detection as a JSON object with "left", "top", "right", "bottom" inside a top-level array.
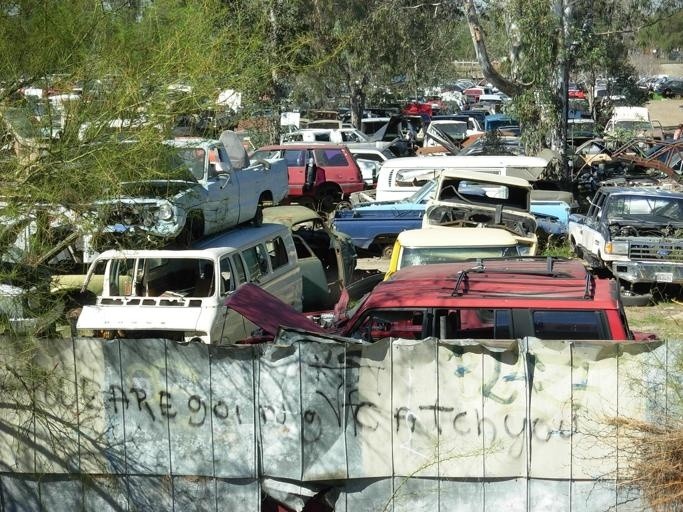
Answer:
[{"left": 193, "top": 277, "right": 224, "bottom": 297}]
